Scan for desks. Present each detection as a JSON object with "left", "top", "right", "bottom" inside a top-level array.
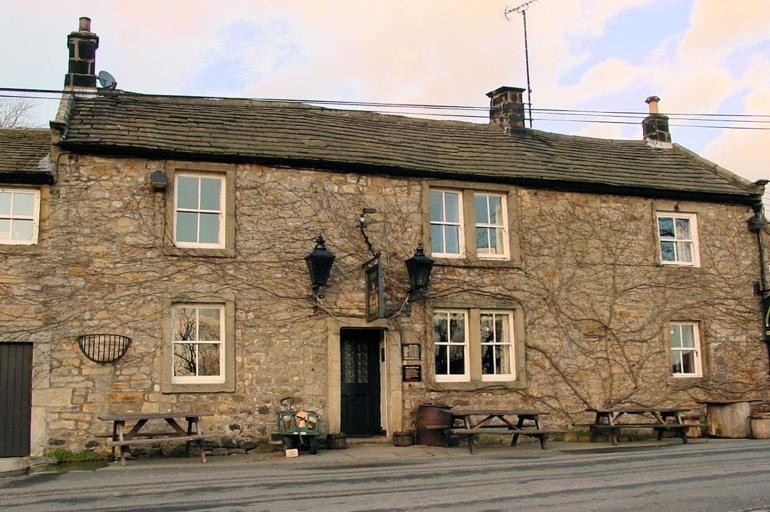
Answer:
[
  {"left": 98, "top": 412, "right": 216, "bottom": 467},
  {"left": 695, "top": 398, "right": 763, "bottom": 439}
]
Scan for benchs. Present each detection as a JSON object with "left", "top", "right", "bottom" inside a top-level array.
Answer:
[
  {"left": 421, "top": 405, "right": 695, "bottom": 456},
  {"left": 94, "top": 431, "right": 204, "bottom": 457},
  {"left": 103, "top": 435, "right": 220, "bottom": 463}
]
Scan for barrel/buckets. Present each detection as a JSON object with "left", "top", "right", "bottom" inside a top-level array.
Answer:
[{"left": 416, "top": 402, "right": 454, "bottom": 447}]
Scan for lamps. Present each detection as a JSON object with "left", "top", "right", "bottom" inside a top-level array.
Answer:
[
  {"left": 405, "top": 240, "right": 434, "bottom": 300},
  {"left": 305, "top": 233, "right": 336, "bottom": 302}
]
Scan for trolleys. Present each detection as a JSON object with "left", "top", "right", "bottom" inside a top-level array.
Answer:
[{"left": 271, "top": 396, "right": 321, "bottom": 454}]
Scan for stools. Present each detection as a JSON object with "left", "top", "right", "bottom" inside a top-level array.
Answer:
[
  {"left": 747, "top": 412, "right": 770, "bottom": 438},
  {"left": 681, "top": 415, "right": 702, "bottom": 438}
]
